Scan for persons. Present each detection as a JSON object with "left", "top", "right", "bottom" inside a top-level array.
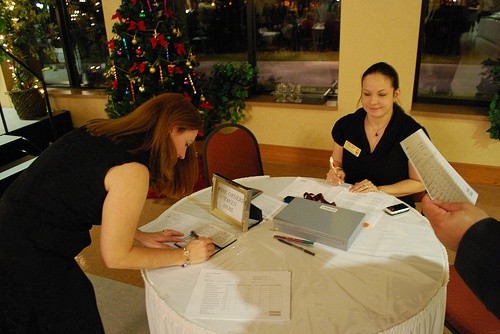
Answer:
[
  {"left": 0, "top": 94, "right": 215, "bottom": 333},
  {"left": 420, "top": 194, "right": 500, "bottom": 323},
  {"left": 326, "top": 61, "right": 429, "bottom": 209}
]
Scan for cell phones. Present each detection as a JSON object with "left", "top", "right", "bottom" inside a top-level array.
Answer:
[{"left": 385, "top": 202, "right": 410, "bottom": 215}]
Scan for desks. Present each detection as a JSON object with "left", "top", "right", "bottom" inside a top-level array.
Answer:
[{"left": 141, "top": 176, "right": 449, "bottom": 334}]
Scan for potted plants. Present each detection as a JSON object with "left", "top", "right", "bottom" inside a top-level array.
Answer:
[{"left": 0, "top": 0, "right": 60, "bottom": 120}]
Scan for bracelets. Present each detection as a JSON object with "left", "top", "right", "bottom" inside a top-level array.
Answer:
[{"left": 181, "top": 245, "right": 190, "bottom": 267}]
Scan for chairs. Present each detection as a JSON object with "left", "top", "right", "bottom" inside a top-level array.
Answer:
[
  {"left": 204, "top": 123, "right": 264, "bottom": 188},
  {"left": 443, "top": 265, "right": 500, "bottom": 334}
]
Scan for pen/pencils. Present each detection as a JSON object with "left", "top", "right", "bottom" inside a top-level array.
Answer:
[
  {"left": 273, "top": 235, "right": 313, "bottom": 246},
  {"left": 190, "top": 230, "right": 198, "bottom": 238},
  {"left": 330, "top": 157, "right": 343, "bottom": 186},
  {"left": 278, "top": 239, "right": 315, "bottom": 256}
]
